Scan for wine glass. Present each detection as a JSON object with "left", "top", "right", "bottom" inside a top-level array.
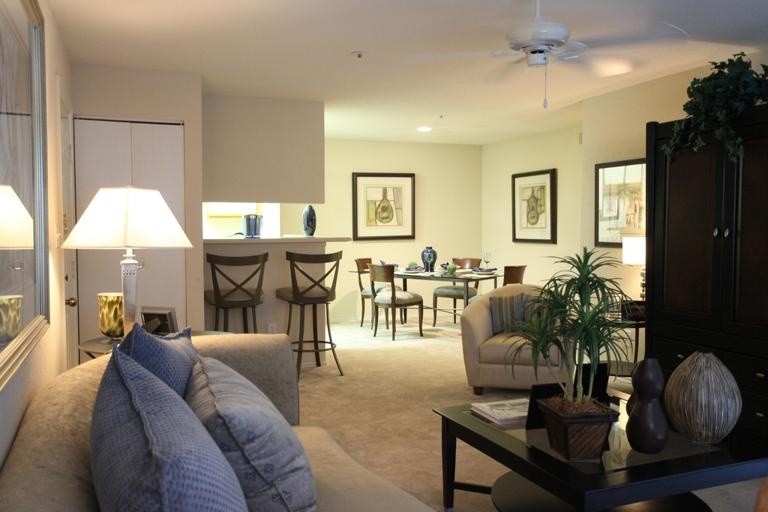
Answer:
[{"left": 483, "top": 250, "right": 491, "bottom": 267}]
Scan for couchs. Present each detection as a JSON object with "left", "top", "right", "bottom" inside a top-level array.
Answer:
[
  {"left": 460, "top": 284, "right": 579, "bottom": 394},
  {"left": 1, "top": 333, "right": 434, "bottom": 512}
]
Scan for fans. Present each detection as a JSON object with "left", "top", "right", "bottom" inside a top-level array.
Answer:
[{"left": 424, "top": 0, "right": 700, "bottom": 110}]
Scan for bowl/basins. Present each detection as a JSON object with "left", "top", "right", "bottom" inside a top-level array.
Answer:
[{"left": 452, "top": 258, "right": 481, "bottom": 268}]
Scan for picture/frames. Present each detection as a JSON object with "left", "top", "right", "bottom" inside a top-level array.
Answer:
[
  {"left": 140, "top": 305, "right": 179, "bottom": 336},
  {"left": 511, "top": 168, "right": 557, "bottom": 244},
  {"left": 352, "top": 172, "right": 416, "bottom": 241},
  {"left": 594, "top": 158, "right": 649, "bottom": 248}
]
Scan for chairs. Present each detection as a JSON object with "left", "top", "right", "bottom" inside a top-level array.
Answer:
[
  {"left": 275, "top": 250, "right": 344, "bottom": 380},
  {"left": 203, "top": 253, "right": 269, "bottom": 333},
  {"left": 354, "top": 258, "right": 527, "bottom": 340}
]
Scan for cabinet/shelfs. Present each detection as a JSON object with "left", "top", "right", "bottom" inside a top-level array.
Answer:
[{"left": 644, "top": 103, "right": 767, "bottom": 436}]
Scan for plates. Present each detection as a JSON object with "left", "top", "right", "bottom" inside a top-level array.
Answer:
[
  {"left": 456, "top": 270, "right": 472, "bottom": 273},
  {"left": 474, "top": 271, "right": 494, "bottom": 275},
  {"left": 403, "top": 271, "right": 421, "bottom": 274}
]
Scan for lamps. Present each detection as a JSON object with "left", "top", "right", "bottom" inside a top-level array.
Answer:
[
  {"left": 0, "top": 185, "right": 35, "bottom": 252},
  {"left": 61, "top": 187, "right": 194, "bottom": 339},
  {"left": 621, "top": 236, "right": 648, "bottom": 301}
]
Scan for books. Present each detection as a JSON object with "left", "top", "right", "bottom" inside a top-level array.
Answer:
[{"left": 470, "top": 398, "right": 529, "bottom": 425}]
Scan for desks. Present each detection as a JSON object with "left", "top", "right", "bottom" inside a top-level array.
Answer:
[
  {"left": 595, "top": 318, "right": 646, "bottom": 378},
  {"left": 77, "top": 330, "right": 234, "bottom": 359}
]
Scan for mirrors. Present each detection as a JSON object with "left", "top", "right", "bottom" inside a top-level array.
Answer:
[{"left": 0, "top": 0, "right": 51, "bottom": 390}]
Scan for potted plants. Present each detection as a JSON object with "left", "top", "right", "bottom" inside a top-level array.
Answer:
[{"left": 507, "top": 248, "right": 649, "bottom": 462}]
